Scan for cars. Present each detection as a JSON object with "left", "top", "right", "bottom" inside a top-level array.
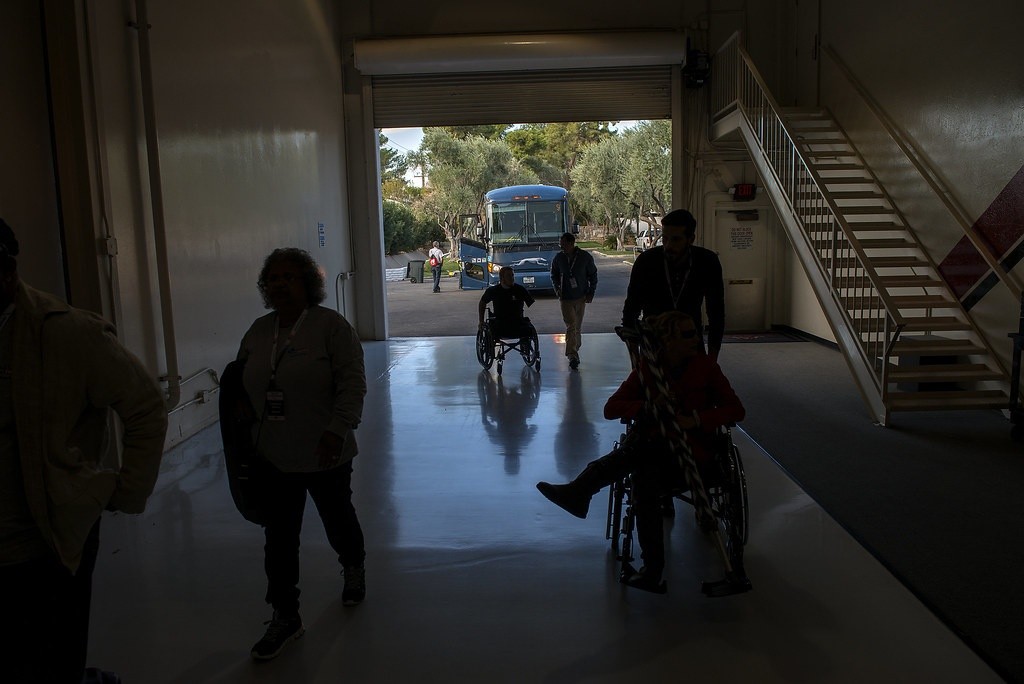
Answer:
[{"left": 636, "top": 228, "right": 663, "bottom": 252}]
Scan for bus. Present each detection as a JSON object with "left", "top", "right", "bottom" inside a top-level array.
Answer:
[{"left": 457, "top": 184, "right": 579, "bottom": 289}]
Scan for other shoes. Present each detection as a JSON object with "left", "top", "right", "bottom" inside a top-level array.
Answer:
[
  {"left": 537, "top": 479, "right": 590, "bottom": 519},
  {"left": 626, "top": 565, "right": 667, "bottom": 596},
  {"left": 567, "top": 352, "right": 581, "bottom": 369}
]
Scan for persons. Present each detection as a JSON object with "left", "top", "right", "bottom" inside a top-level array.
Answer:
[
  {"left": 537, "top": 313, "right": 746, "bottom": 591},
  {"left": 219, "top": 246, "right": 368, "bottom": 658},
  {"left": 622, "top": 209, "right": 724, "bottom": 374},
  {"left": 477, "top": 267, "right": 534, "bottom": 332},
  {"left": 550, "top": 233, "right": 599, "bottom": 367},
  {"left": 0, "top": 218, "right": 168, "bottom": 683},
  {"left": 429, "top": 241, "right": 443, "bottom": 292}
]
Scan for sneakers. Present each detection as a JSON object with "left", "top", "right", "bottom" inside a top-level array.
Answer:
[
  {"left": 340, "top": 564, "right": 366, "bottom": 608},
  {"left": 251, "top": 609, "right": 305, "bottom": 659}
]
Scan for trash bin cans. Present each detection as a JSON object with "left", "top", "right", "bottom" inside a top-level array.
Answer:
[{"left": 403, "top": 260, "right": 425, "bottom": 284}]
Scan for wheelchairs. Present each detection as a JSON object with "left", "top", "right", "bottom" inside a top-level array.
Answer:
[
  {"left": 476, "top": 308, "right": 540, "bottom": 374},
  {"left": 606, "top": 422, "right": 750, "bottom": 581}
]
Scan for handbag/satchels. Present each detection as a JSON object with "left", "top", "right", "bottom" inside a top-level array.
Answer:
[{"left": 430, "top": 257, "right": 438, "bottom": 266}]
